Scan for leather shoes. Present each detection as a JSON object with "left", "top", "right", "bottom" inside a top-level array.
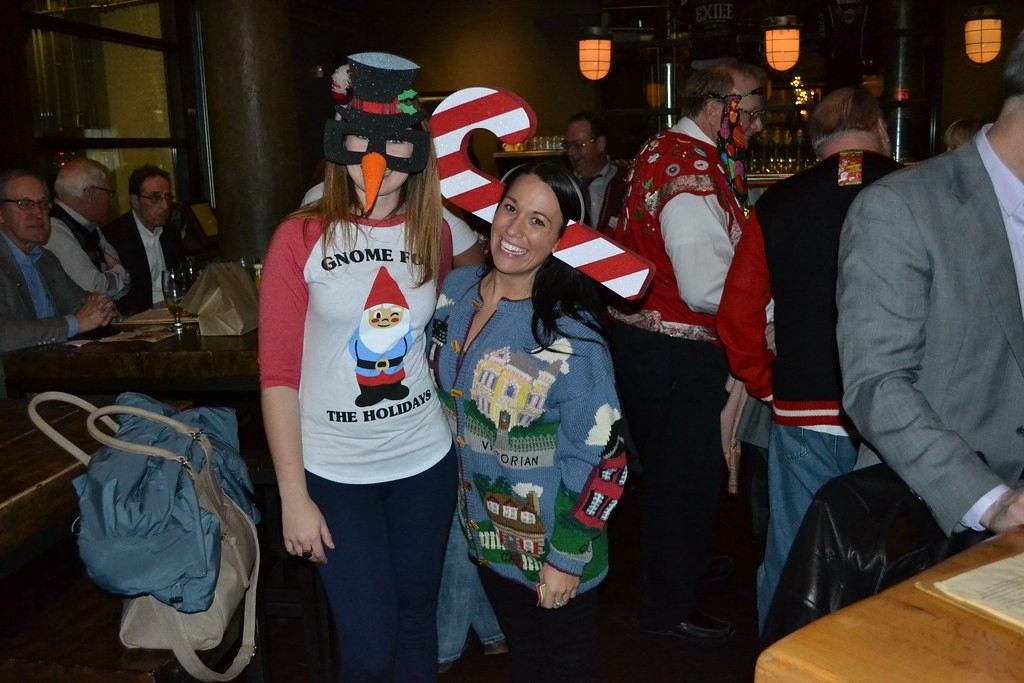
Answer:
[
  {"left": 697, "top": 553, "right": 737, "bottom": 582},
  {"left": 642, "top": 606, "right": 737, "bottom": 645}
]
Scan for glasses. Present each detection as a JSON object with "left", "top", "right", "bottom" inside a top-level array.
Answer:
[
  {"left": 3, "top": 198, "right": 57, "bottom": 213},
  {"left": 134, "top": 190, "right": 176, "bottom": 203},
  {"left": 561, "top": 137, "right": 595, "bottom": 152},
  {"left": 83, "top": 185, "right": 117, "bottom": 198},
  {"left": 717, "top": 99, "right": 766, "bottom": 123}
]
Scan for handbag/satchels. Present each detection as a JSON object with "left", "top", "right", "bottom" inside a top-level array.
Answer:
[{"left": 27, "top": 389, "right": 261, "bottom": 683}]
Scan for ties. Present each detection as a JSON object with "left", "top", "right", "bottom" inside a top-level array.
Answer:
[{"left": 571, "top": 173, "right": 602, "bottom": 227}]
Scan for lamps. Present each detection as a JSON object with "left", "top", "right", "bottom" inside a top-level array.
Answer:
[
  {"left": 762, "top": 14, "right": 801, "bottom": 70},
  {"left": 965, "top": 0, "right": 1002, "bottom": 62},
  {"left": 577, "top": 26, "right": 611, "bottom": 79}
]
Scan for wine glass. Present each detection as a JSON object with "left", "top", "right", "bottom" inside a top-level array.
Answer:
[{"left": 160, "top": 261, "right": 197, "bottom": 334}]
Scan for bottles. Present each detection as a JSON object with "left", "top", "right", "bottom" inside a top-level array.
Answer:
[
  {"left": 253, "top": 263, "right": 263, "bottom": 290},
  {"left": 744, "top": 127, "right": 806, "bottom": 173}
]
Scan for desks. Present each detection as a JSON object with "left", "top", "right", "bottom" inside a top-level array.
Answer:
[
  {"left": 753, "top": 528, "right": 1024, "bottom": 683},
  {"left": 0, "top": 295, "right": 260, "bottom": 391}
]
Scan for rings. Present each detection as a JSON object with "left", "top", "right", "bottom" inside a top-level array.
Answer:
[
  {"left": 555, "top": 601, "right": 565, "bottom": 607},
  {"left": 302, "top": 547, "right": 313, "bottom": 559}
]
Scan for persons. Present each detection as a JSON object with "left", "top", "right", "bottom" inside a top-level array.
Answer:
[
  {"left": 255, "top": 57, "right": 516, "bottom": 683},
  {"left": 0, "top": 165, "right": 120, "bottom": 355},
  {"left": 837, "top": 48, "right": 1024, "bottom": 533},
  {"left": 555, "top": 111, "right": 633, "bottom": 324},
  {"left": 717, "top": 86, "right": 905, "bottom": 640},
  {"left": 424, "top": 158, "right": 629, "bottom": 683},
  {"left": 99, "top": 162, "right": 206, "bottom": 319},
  {"left": 598, "top": 53, "right": 765, "bottom": 640},
  {"left": 42, "top": 156, "right": 132, "bottom": 303}
]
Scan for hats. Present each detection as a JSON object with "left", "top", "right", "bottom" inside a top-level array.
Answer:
[{"left": 322, "top": 53, "right": 430, "bottom": 129}]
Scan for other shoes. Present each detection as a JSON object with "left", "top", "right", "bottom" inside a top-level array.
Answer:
[
  {"left": 478, "top": 647, "right": 513, "bottom": 682},
  {"left": 438, "top": 658, "right": 460, "bottom": 683}
]
